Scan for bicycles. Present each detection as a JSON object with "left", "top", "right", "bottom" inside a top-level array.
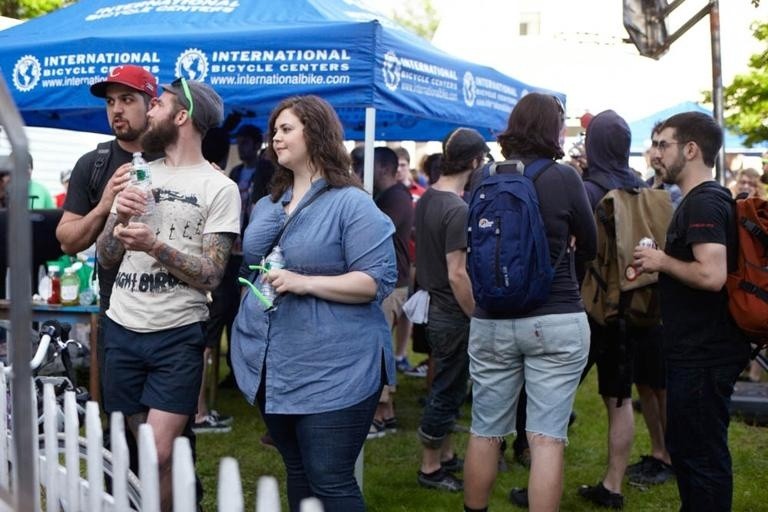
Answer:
[{"left": 0, "top": 320, "right": 142, "bottom": 511}]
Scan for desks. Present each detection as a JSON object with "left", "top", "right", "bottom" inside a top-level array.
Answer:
[{"left": 0, "top": 300, "right": 102, "bottom": 400}]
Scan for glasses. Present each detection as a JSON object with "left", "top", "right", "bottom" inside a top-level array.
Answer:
[
  {"left": 238, "top": 265, "right": 289, "bottom": 314},
  {"left": 170, "top": 75, "right": 194, "bottom": 119}
]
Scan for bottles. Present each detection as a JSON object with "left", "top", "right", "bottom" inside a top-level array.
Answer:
[
  {"left": 45, "top": 265, "right": 61, "bottom": 304},
  {"left": 259, "top": 246, "right": 284, "bottom": 311},
  {"left": 58, "top": 261, "right": 82, "bottom": 305},
  {"left": 132, "top": 151, "right": 155, "bottom": 213}
]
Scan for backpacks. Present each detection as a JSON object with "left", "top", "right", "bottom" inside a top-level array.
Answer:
[
  {"left": 464, "top": 158, "right": 568, "bottom": 312},
  {"left": 668, "top": 186, "right": 768, "bottom": 345},
  {"left": 580, "top": 171, "right": 674, "bottom": 329}
]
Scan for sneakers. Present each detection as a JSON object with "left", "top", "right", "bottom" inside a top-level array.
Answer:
[
  {"left": 396, "top": 355, "right": 429, "bottom": 377},
  {"left": 624, "top": 455, "right": 674, "bottom": 487},
  {"left": 578, "top": 483, "right": 623, "bottom": 510},
  {"left": 498, "top": 450, "right": 529, "bottom": 507},
  {"left": 366, "top": 416, "right": 402, "bottom": 439},
  {"left": 417, "top": 383, "right": 465, "bottom": 494},
  {"left": 191, "top": 411, "right": 232, "bottom": 436}
]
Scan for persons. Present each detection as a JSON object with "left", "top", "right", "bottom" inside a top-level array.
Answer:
[
  {"left": 351, "top": 129, "right": 496, "bottom": 492},
  {"left": 464, "top": 94, "right": 599, "bottom": 511},
  {"left": 25, "top": 150, "right": 56, "bottom": 211},
  {"left": 230, "top": 96, "right": 397, "bottom": 512},
  {"left": 723, "top": 153, "right": 768, "bottom": 381},
  {"left": 0, "top": 151, "right": 15, "bottom": 211},
  {"left": 54, "top": 166, "right": 74, "bottom": 210},
  {"left": 56, "top": 64, "right": 268, "bottom": 511},
  {"left": 500, "top": 110, "right": 680, "bottom": 512},
  {"left": 633, "top": 112, "right": 739, "bottom": 512}
]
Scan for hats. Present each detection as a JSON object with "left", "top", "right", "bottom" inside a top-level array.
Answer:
[
  {"left": 159, "top": 80, "right": 224, "bottom": 130},
  {"left": 443, "top": 127, "right": 485, "bottom": 160},
  {"left": 90, "top": 64, "right": 157, "bottom": 98}
]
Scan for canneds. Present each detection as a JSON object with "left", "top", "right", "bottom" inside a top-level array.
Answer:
[{"left": 638, "top": 236, "right": 658, "bottom": 251}]
